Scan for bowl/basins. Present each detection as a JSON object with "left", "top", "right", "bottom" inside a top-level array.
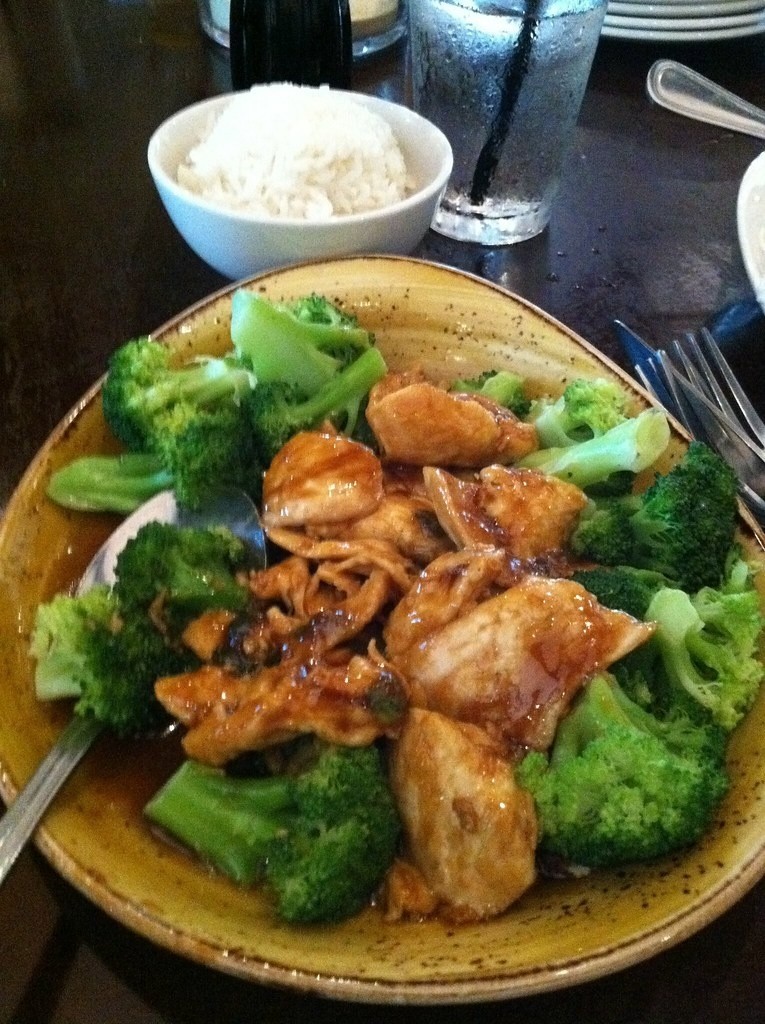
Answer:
[{"left": 147, "top": 82, "right": 455, "bottom": 280}]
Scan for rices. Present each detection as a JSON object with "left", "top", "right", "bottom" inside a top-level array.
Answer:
[{"left": 176, "top": 80, "right": 414, "bottom": 218}]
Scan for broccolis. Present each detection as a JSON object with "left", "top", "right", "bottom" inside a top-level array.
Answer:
[
  {"left": 445, "top": 364, "right": 764, "bottom": 875},
  {"left": 25, "top": 285, "right": 407, "bottom": 925}
]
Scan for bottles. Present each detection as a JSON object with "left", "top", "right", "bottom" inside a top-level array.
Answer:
[{"left": 229, "top": 0, "right": 353, "bottom": 92}]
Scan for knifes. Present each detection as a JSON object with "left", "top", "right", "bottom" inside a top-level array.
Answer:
[{"left": 612, "top": 320, "right": 765, "bottom": 510}]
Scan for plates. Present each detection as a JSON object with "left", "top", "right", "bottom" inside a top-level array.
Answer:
[
  {"left": 738, "top": 150, "right": 765, "bottom": 313},
  {"left": 600, "top": 0, "right": 765, "bottom": 42},
  {"left": 198, "top": 7, "right": 406, "bottom": 57},
  {"left": 0, "top": 254, "right": 765, "bottom": 1005}
]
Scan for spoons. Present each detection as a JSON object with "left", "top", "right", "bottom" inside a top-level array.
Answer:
[{"left": 0, "top": 480, "right": 266, "bottom": 905}]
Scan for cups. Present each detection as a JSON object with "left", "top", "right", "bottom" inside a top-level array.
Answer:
[{"left": 405, "top": 0, "right": 607, "bottom": 246}]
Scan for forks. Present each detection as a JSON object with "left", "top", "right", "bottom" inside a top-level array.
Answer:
[{"left": 634, "top": 326, "right": 765, "bottom": 517}]
